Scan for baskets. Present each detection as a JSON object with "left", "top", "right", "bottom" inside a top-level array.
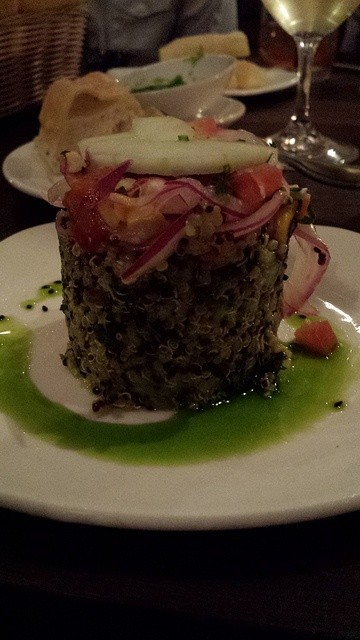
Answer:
[{"left": 1, "top": 1, "right": 90, "bottom": 118}]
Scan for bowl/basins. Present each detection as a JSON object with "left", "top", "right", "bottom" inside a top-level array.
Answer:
[{"left": 119, "top": 52, "right": 239, "bottom": 118}]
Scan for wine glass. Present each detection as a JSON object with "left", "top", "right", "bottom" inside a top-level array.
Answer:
[{"left": 258, "top": 1, "right": 360, "bottom": 166}]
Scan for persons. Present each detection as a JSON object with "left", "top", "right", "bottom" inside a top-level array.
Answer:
[{"left": 79, "top": 0, "right": 239, "bottom": 69}]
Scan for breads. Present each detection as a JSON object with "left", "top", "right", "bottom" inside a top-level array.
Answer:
[{"left": 38, "top": 64, "right": 140, "bottom": 173}]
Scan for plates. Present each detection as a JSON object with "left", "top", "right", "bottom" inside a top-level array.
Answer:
[
  {"left": 215, "top": 67, "right": 303, "bottom": 105},
  {"left": 2, "top": 137, "right": 283, "bottom": 208},
  {"left": 0, "top": 222, "right": 359, "bottom": 532},
  {"left": 175, "top": 94, "right": 246, "bottom": 125}
]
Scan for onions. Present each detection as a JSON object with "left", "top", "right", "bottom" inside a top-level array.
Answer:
[{"left": 54, "top": 166, "right": 326, "bottom": 318}]
